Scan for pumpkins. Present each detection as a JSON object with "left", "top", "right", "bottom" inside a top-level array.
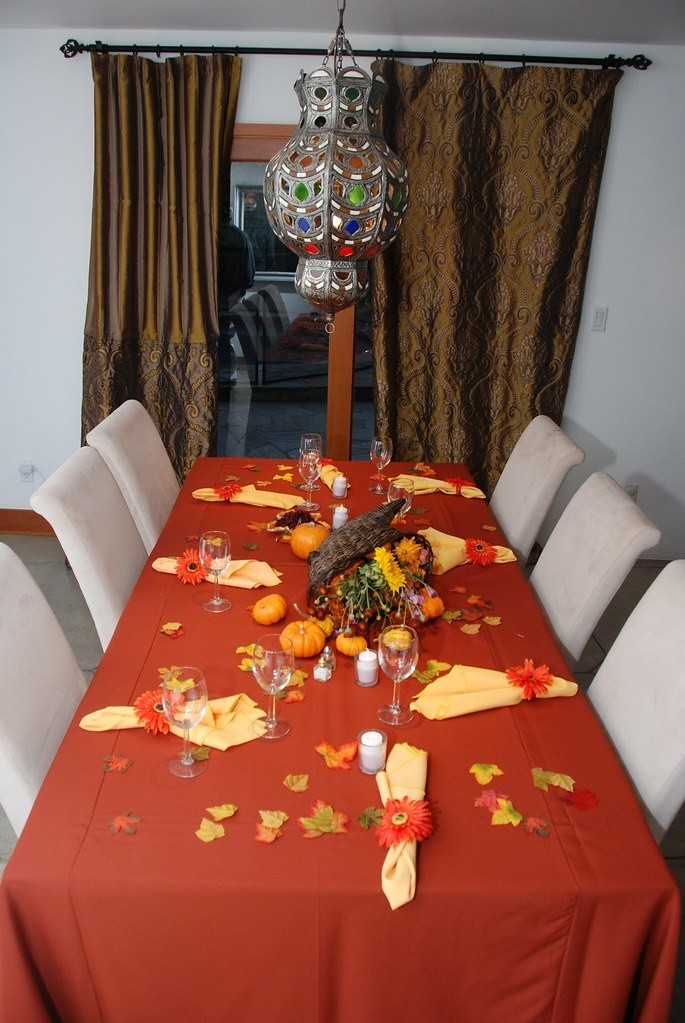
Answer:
[
  {"left": 252, "top": 594, "right": 286, "bottom": 625},
  {"left": 291, "top": 522, "right": 333, "bottom": 558},
  {"left": 279, "top": 601, "right": 368, "bottom": 658},
  {"left": 417, "top": 586, "right": 445, "bottom": 620}
]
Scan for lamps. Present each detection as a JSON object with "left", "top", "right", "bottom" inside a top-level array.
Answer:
[{"left": 262, "top": 1, "right": 408, "bottom": 305}]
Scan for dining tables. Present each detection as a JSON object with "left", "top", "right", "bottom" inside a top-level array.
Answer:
[
  {"left": 0, "top": 456, "right": 681, "bottom": 1022},
  {"left": 263, "top": 311, "right": 329, "bottom": 363}
]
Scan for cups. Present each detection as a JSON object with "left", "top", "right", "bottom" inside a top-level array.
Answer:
[
  {"left": 353, "top": 649, "right": 379, "bottom": 687},
  {"left": 332, "top": 476, "right": 348, "bottom": 499},
  {"left": 331, "top": 506, "right": 351, "bottom": 531},
  {"left": 356, "top": 727, "right": 388, "bottom": 775}
]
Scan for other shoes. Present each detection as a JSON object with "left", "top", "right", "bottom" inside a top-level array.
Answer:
[{"left": 217, "top": 379, "right": 236, "bottom": 389}]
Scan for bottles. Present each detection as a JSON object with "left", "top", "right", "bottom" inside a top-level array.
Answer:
[
  {"left": 313, "top": 657, "right": 332, "bottom": 682},
  {"left": 321, "top": 646, "right": 337, "bottom": 672}
]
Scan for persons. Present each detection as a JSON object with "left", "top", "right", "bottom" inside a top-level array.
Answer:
[{"left": 217, "top": 202, "right": 256, "bottom": 391}]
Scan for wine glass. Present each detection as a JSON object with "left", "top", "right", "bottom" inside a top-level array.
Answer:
[
  {"left": 368, "top": 436, "right": 393, "bottom": 494},
  {"left": 161, "top": 667, "right": 208, "bottom": 779},
  {"left": 251, "top": 634, "right": 294, "bottom": 739},
  {"left": 299, "top": 433, "right": 322, "bottom": 492},
  {"left": 387, "top": 477, "right": 414, "bottom": 524},
  {"left": 198, "top": 530, "right": 232, "bottom": 614},
  {"left": 298, "top": 449, "right": 322, "bottom": 511},
  {"left": 378, "top": 623, "right": 419, "bottom": 726}
]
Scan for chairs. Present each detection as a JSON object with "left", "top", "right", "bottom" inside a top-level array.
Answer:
[
  {"left": 0, "top": 397, "right": 684, "bottom": 1023},
  {"left": 229, "top": 283, "right": 290, "bottom": 399}
]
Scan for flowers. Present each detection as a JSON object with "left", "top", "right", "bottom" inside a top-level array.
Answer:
[
  {"left": 446, "top": 477, "right": 476, "bottom": 486},
  {"left": 506, "top": 656, "right": 553, "bottom": 699},
  {"left": 175, "top": 548, "right": 212, "bottom": 586},
  {"left": 357, "top": 796, "right": 434, "bottom": 846},
  {"left": 317, "top": 457, "right": 335, "bottom": 465},
  {"left": 133, "top": 689, "right": 186, "bottom": 734},
  {"left": 465, "top": 537, "right": 498, "bottom": 567},
  {"left": 215, "top": 483, "right": 243, "bottom": 499},
  {"left": 316, "top": 535, "right": 439, "bottom": 636}
]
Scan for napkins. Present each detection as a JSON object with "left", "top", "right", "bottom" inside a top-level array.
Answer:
[
  {"left": 388, "top": 473, "right": 487, "bottom": 499},
  {"left": 316, "top": 461, "right": 351, "bottom": 492},
  {"left": 151, "top": 556, "right": 282, "bottom": 590},
  {"left": 376, "top": 742, "right": 427, "bottom": 907},
  {"left": 192, "top": 484, "right": 306, "bottom": 510},
  {"left": 79, "top": 694, "right": 267, "bottom": 752},
  {"left": 408, "top": 663, "right": 578, "bottom": 720},
  {"left": 416, "top": 528, "right": 517, "bottom": 575}
]
